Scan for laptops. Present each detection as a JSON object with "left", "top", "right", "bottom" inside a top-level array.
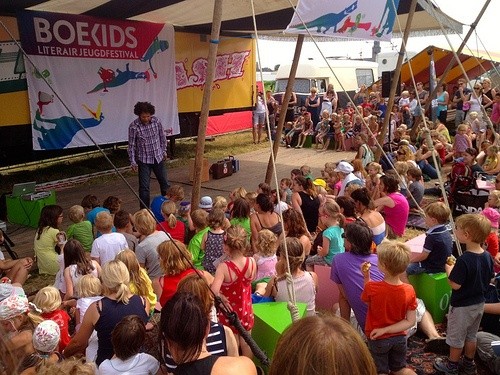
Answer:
[{"left": 5, "top": 182, "right": 36, "bottom": 198}]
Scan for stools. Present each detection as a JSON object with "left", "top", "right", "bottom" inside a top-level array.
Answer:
[
  {"left": 410, "top": 273, "right": 454, "bottom": 325},
  {"left": 285, "top": 130, "right": 335, "bottom": 150},
  {"left": 5, "top": 192, "right": 55, "bottom": 228},
  {"left": 249, "top": 301, "right": 307, "bottom": 374}
]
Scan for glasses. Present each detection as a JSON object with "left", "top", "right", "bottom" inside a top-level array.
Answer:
[
  {"left": 342, "top": 233, "right": 347, "bottom": 238},
  {"left": 474, "top": 88, "right": 479, "bottom": 90}
]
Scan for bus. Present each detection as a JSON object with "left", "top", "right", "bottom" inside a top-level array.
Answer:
[{"left": 272, "top": 62, "right": 378, "bottom": 117}]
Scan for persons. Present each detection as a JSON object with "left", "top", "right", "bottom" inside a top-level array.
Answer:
[
  {"left": 0, "top": 78, "right": 500, "bottom": 375},
  {"left": 252, "top": 93, "right": 266, "bottom": 144},
  {"left": 128, "top": 101, "right": 171, "bottom": 209}
]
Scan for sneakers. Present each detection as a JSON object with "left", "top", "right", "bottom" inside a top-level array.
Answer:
[
  {"left": 434, "top": 357, "right": 459, "bottom": 375},
  {"left": 459, "top": 355, "right": 473, "bottom": 374}
]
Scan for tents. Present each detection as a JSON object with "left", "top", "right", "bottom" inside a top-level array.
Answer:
[{"left": 0, "top": 0, "right": 490, "bottom": 213}]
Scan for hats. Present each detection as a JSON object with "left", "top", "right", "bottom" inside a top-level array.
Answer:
[
  {"left": 334, "top": 162, "right": 354, "bottom": 173},
  {"left": 0, "top": 294, "right": 29, "bottom": 321},
  {"left": 313, "top": 178, "right": 326, "bottom": 187},
  {"left": 199, "top": 196, "right": 213, "bottom": 208},
  {"left": 33, "top": 320, "right": 61, "bottom": 352}
]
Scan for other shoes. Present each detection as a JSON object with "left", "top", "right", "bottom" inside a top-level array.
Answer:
[{"left": 427, "top": 337, "right": 451, "bottom": 355}]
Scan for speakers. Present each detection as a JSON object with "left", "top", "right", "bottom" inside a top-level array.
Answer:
[
  {"left": 382, "top": 71, "right": 401, "bottom": 97},
  {"left": 213, "top": 161, "right": 232, "bottom": 179}
]
